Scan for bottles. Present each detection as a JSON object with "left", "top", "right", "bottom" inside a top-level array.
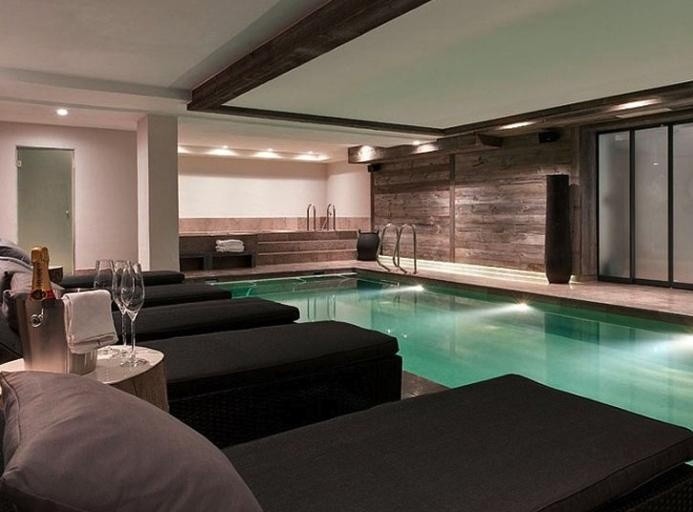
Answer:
[{"left": 26, "top": 246, "right": 55, "bottom": 300}]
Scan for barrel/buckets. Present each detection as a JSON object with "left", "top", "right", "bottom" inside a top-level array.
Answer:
[{"left": 15, "top": 288, "right": 98, "bottom": 377}]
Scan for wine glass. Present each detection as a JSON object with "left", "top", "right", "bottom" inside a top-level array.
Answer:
[{"left": 94, "top": 259, "right": 146, "bottom": 369}]
